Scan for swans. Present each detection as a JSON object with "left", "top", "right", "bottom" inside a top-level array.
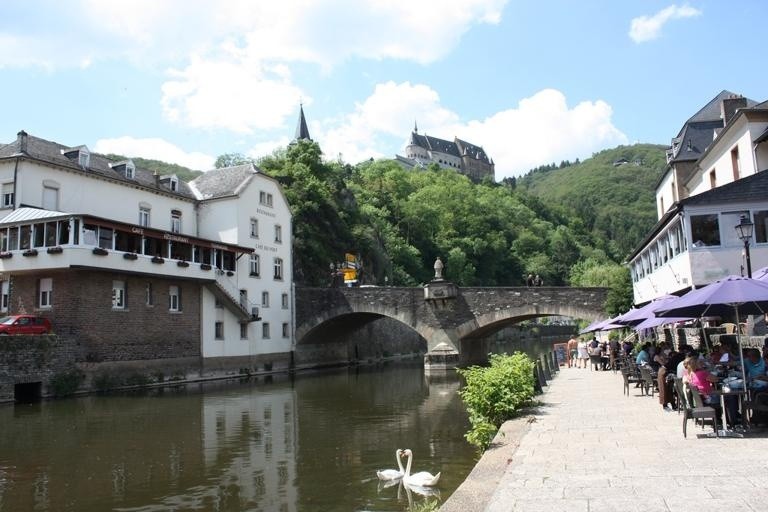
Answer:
[
  {"left": 401, "top": 449, "right": 441, "bottom": 487},
  {"left": 403, "top": 484, "right": 441, "bottom": 512},
  {"left": 377, "top": 479, "right": 404, "bottom": 502},
  {"left": 376, "top": 448, "right": 405, "bottom": 481}
]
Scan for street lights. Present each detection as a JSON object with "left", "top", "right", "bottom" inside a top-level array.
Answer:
[
  {"left": 736, "top": 212, "right": 755, "bottom": 278},
  {"left": 328, "top": 260, "right": 345, "bottom": 286}
]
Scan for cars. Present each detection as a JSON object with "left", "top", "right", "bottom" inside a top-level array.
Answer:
[{"left": 0, "top": 315, "right": 52, "bottom": 336}]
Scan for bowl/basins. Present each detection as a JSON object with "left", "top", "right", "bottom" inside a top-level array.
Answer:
[{"left": 723, "top": 376, "right": 767, "bottom": 388}]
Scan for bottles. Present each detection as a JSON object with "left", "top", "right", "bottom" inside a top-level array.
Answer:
[{"left": 721, "top": 383, "right": 730, "bottom": 392}]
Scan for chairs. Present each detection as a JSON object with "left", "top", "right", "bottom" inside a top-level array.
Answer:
[{"left": 588, "top": 350, "right": 768, "bottom": 439}]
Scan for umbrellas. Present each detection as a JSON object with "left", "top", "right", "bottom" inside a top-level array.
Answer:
[{"left": 577, "top": 266, "right": 768, "bottom": 422}]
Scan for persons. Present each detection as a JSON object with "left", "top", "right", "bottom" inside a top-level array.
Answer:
[
  {"left": 528, "top": 272, "right": 534, "bottom": 287},
  {"left": 567, "top": 335, "right": 768, "bottom": 434},
  {"left": 534, "top": 274, "right": 544, "bottom": 286},
  {"left": 434, "top": 256, "right": 444, "bottom": 278}
]
